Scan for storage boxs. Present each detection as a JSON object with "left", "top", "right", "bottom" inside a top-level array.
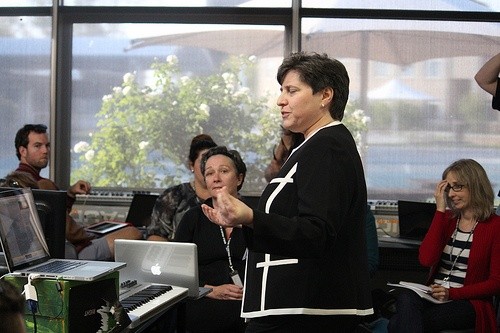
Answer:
[{"left": 4, "top": 271, "right": 120, "bottom": 333}]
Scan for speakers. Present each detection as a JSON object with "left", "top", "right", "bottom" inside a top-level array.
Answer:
[{"left": 3, "top": 271, "right": 120, "bottom": 333}]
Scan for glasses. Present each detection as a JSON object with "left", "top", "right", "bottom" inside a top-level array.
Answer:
[{"left": 445, "top": 185, "right": 464, "bottom": 192}]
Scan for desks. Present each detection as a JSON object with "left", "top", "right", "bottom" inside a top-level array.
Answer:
[{"left": 378, "top": 241, "right": 425, "bottom": 271}]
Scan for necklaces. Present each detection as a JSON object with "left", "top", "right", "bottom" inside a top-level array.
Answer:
[{"left": 192, "top": 180, "right": 202, "bottom": 206}]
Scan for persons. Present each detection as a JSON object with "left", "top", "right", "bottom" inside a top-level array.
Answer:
[
  {"left": 388, "top": 159, "right": 500, "bottom": 333},
  {"left": 147, "top": 134, "right": 218, "bottom": 242},
  {"left": 175, "top": 145, "right": 253, "bottom": 332},
  {"left": 0, "top": 124, "right": 143, "bottom": 262},
  {"left": 202, "top": 50, "right": 379, "bottom": 333},
  {"left": 475, "top": 52, "right": 500, "bottom": 111}
]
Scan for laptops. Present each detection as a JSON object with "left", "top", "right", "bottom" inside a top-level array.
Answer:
[
  {"left": 125, "top": 193, "right": 160, "bottom": 227},
  {"left": 113, "top": 238, "right": 213, "bottom": 302},
  {"left": 398, "top": 200, "right": 437, "bottom": 241},
  {"left": 0, "top": 186, "right": 127, "bottom": 282}
]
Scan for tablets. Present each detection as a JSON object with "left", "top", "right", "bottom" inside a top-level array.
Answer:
[{"left": 84, "top": 219, "right": 129, "bottom": 234}]
